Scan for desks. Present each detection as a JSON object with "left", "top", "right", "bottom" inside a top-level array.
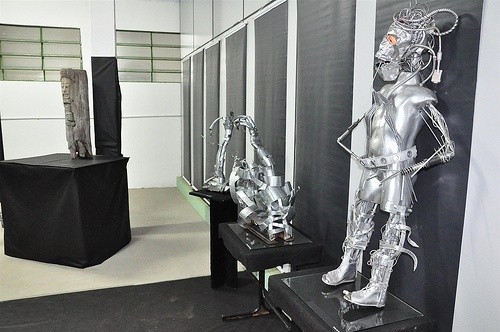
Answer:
[
  {"left": 189, "top": 190, "right": 238, "bottom": 290},
  {"left": 266, "top": 265, "right": 429, "bottom": 332},
  {"left": 218, "top": 219, "right": 322, "bottom": 322},
  {"left": 0, "top": 153, "right": 131, "bottom": 268}
]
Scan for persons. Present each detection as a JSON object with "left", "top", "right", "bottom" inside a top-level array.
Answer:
[
  {"left": 321, "top": 283, "right": 383, "bottom": 332},
  {"left": 321, "top": 0, "right": 459, "bottom": 307}
]
